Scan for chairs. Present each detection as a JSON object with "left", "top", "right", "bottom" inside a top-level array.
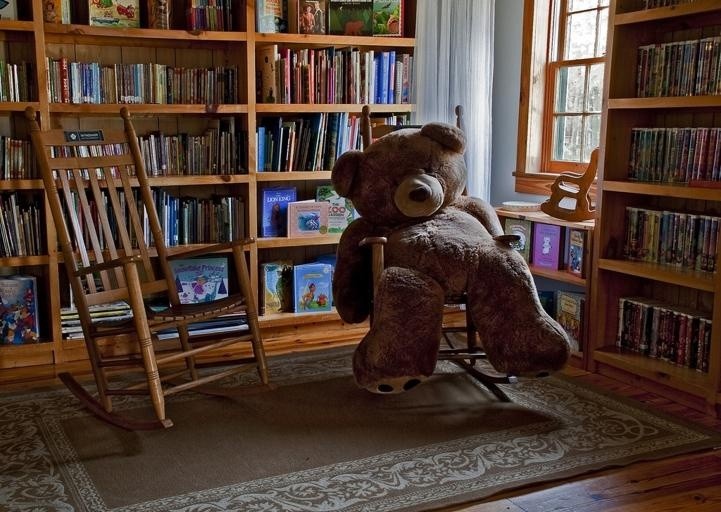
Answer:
[
  {"left": 541, "top": 147, "right": 598, "bottom": 222},
  {"left": 357, "top": 99, "right": 524, "bottom": 392},
  {"left": 24, "top": 103, "right": 274, "bottom": 434}
]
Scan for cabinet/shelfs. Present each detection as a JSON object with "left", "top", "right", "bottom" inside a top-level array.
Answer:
[
  {"left": 42, "top": 0, "right": 251, "bottom": 364},
  {"left": 498, "top": 207, "right": 591, "bottom": 375},
  {"left": 584, "top": 0, "right": 720, "bottom": 415},
  {"left": 252, "top": 0, "right": 422, "bottom": 327},
  {"left": 0, "top": 0, "right": 52, "bottom": 368}
]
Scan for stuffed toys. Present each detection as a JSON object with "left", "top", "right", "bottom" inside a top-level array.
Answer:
[{"left": 330, "top": 121, "right": 571, "bottom": 394}]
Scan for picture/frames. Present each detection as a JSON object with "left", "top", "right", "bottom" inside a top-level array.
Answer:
[{"left": 296, "top": 0, "right": 329, "bottom": 34}]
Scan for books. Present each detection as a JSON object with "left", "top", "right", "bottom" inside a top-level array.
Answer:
[
  {"left": 46, "top": 57, "right": 238, "bottom": 104},
  {"left": 624, "top": 207, "right": 721, "bottom": 273},
  {"left": 261, "top": 186, "right": 354, "bottom": 237},
  {"left": 537, "top": 289, "right": 586, "bottom": 352},
  {"left": 0, "top": 192, "right": 44, "bottom": 257},
  {"left": 60, "top": 188, "right": 245, "bottom": 250},
  {"left": 0, "top": 60, "right": 34, "bottom": 102},
  {"left": 628, "top": 127, "right": 721, "bottom": 186},
  {"left": 635, "top": 37, "right": 721, "bottom": 97},
  {"left": 0, "top": 135, "right": 41, "bottom": 179},
  {"left": 43, "top": 0, "right": 233, "bottom": 31},
  {"left": 51, "top": 117, "right": 244, "bottom": 180},
  {"left": 0, "top": 0, "right": 18, "bottom": 20},
  {"left": 617, "top": 297, "right": 712, "bottom": 373},
  {"left": 0, "top": 275, "right": 40, "bottom": 344},
  {"left": 505, "top": 219, "right": 587, "bottom": 278},
  {"left": 260, "top": 255, "right": 336, "bottom": 315},
  {"left": 60, "top": 258, "right": 248, "bottom": 340},
  {"left": 256, "top": 112, "right": 410, "bottom": 172},
  {"left": 643, "top": 0, "right": 694, "bottom": 10},
  {"left": 256, "top": 1, "right": 404, "bottom": 38},
  {"left": 256, "top": 44, "right": 413, "bottom": 104}
]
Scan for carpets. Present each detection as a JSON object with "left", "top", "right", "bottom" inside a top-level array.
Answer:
[{"left": 35, "top": 324, "right": 719, "bottom": 510}]
